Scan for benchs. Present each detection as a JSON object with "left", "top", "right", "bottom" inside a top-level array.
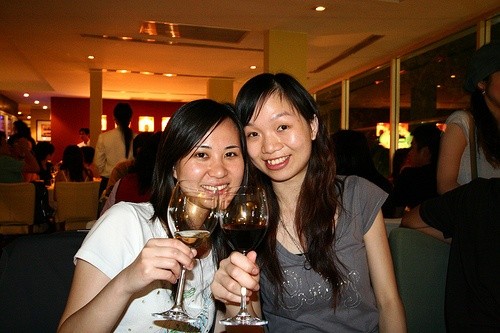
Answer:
[{"left": 0, "top": 222, "right": 400, "bottom": 333}]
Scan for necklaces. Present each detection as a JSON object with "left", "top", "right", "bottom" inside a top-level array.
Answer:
[{"left": 278, "top": 216, "right": 313, "bottom": 271}]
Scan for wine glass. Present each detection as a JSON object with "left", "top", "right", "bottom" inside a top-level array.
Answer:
[
  {"left": 217, "top": 184, "right": 269, "bottom": 325},
  {"left": 150, "top": 178, "right": 221, "bottom": 322}
]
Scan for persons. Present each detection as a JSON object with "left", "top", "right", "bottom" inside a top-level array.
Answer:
[
  {"left": 436, "top": 40, "right": 500, "bottom": 196},
  {"left": 209, "top": 71, "right": 407, "bottom": 333},
  {"left": 92, "top": 101, "right": 139, "bottom": 222},
  {"left": 0, "top": 119, "right": 95, "bottom": 226},
  {"left": 400, "top": 177, "right": 500, "bottom": 333},
  {"left": 56, "top": 97, "right": 250, "bottom": 333},
  {"left": 387, "top": 122, "right": 442, "bottom": 217}
]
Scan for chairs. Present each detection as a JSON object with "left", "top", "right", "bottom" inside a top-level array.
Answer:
[
  {"left": 55, "top": 182, "right": 99, "bottom": 230},
  {"left": 0, "top": 183, "right": 35, "bottom": 232}
]
